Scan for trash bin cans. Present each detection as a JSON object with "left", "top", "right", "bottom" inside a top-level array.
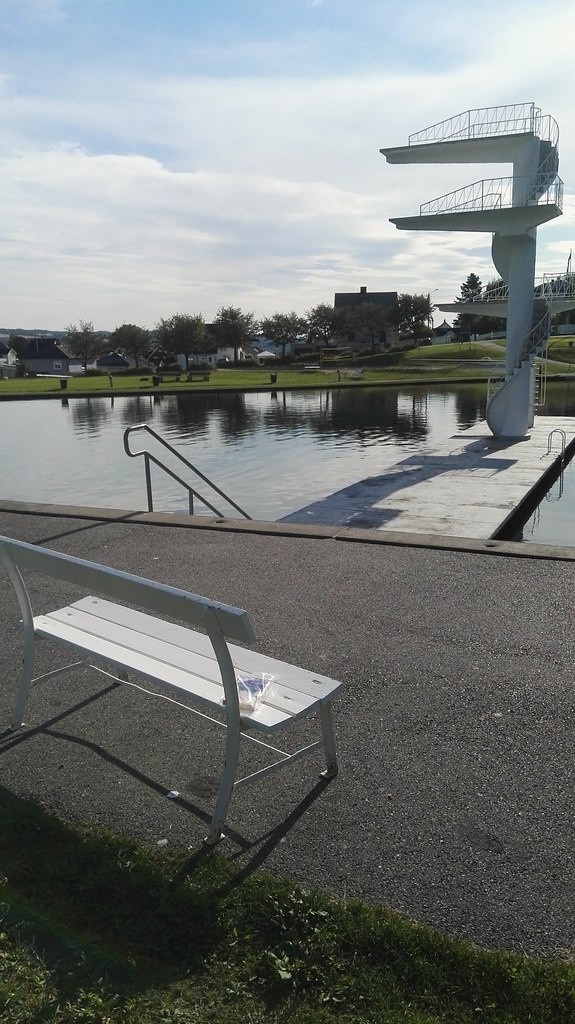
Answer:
[
  {"left": 61, "top": 379, "right": 67, "bottom": 390},
  {"left": 62, "top": 397, "right": 68, "bottom": 407},
  {"left": 154, "top": 395, "right": 161, "bottom": 406},
  {"left": 271, "top": 374, "right": 277, "bottom": 383},
  {"left": 153, "top": 375, "right": 159, "bottom": 386},
  {"left": 271, "top": 391, "right": 277, "bottom": 400}
]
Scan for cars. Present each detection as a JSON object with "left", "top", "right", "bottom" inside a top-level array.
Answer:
[{"left": 419, "top": 338, "right": 432, "bottom": 346}]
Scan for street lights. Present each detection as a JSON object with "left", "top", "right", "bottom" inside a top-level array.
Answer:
[{"left": 428, "top": 288, "right": 438, "bottom": 327}]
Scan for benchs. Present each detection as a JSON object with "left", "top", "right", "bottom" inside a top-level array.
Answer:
[
  {"left": 185, "top": 370, "right": 211, "bottom": 382},
  {"left": 156, "top": 371, "right": 182, "bottom": 382},
  {"left": 0, "top": 533, "right": 345, "bottom": 847},
  {"left": 304, "top": 362, "right": 322, "bottom": 372}
]
